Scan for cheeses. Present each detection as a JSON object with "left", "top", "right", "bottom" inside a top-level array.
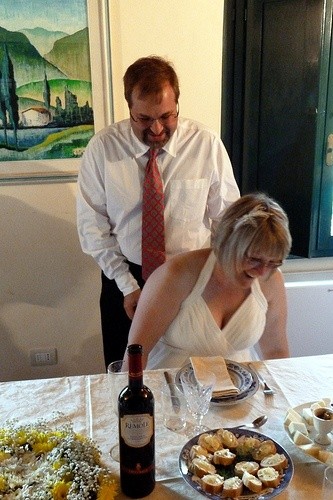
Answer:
[{"left": 285, "top": 398, "right": 333, "bottom": 462}]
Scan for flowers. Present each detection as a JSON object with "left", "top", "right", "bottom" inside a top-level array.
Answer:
[{"left": 0, "top": 409, "right": 120, "bottom": 500}]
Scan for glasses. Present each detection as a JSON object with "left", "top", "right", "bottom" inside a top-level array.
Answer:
[
  {"left": 244, "top": 252, "right": 283, "bottom": 270},
  {"left": 129, "top": 100, "right": 181, "bottom": 127}
]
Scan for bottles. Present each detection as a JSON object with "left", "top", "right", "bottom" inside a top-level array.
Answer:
[{"left": 117, "top": 344, "right": 157, "bottom": 499}]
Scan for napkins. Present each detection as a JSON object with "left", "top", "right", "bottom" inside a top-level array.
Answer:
[{"left": 188, "top": 355, "right": 240, "bottom": 397}]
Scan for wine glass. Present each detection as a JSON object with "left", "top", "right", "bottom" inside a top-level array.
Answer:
[
  {"left": 107, "top": 361, "right": 128, "bottom": 462},
  {"left": 312, "top": 407, "right": 333, "bottom": 446},
  {"left": 180, "top": 368, "right": 216, "bottom": 439}
]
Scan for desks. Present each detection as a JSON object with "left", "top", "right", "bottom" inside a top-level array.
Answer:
[{"left": 0, "top": 353, "right": 333, "bottom": 500}]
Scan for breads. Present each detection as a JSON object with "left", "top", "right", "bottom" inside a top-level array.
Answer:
[{"left": 189, "top": 429, "right": 287, "bottom": 497}]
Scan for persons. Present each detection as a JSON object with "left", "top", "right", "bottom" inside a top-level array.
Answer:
[
  {"left": 75, "top": 57, "right": 241, "bottom": 374},
  {"left": 122, "top": 192, "right": 293, "bottom": 373}
]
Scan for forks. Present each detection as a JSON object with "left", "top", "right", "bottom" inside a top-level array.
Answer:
[{"left": 248, "top": 363, "right": 275, "bottom": 395}]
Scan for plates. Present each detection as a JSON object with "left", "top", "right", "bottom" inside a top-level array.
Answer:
[
  {"left": 283, "top": 401, "right": 333, "bottom": 469},
  {"left": 178, "top": 428, "right": 294, "bottom": 500},
  {"left": 175, "top": 358, "right": 260, "bottom": 406}
]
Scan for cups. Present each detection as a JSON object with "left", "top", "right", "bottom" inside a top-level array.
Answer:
[
  {"left": 160, "top": 382, "right": 188, "bottom": 431},
  {"left": 322, "top": 466, "right": 333, "bottom": 500}
]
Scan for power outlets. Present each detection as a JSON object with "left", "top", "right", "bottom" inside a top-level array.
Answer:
[{"left": 29, "top": 348, "right": 58, "bottom": 366}]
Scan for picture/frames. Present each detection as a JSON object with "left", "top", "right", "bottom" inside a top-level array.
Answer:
[{"left": 0, "top": 0, "right": 116, "bottom": 186}]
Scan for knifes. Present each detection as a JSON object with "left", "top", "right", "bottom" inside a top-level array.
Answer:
[{"left": 164, "top": 371, "right": 181, "bottom": 414}]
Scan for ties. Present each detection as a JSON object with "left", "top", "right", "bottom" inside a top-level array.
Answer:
[{"left": 141, "top": 147, "right": 167, "bottom": 282}]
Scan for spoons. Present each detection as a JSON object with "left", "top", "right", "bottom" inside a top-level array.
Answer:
[{"left": 234, "top": 415, "right": 268, "bottom": 430}]
